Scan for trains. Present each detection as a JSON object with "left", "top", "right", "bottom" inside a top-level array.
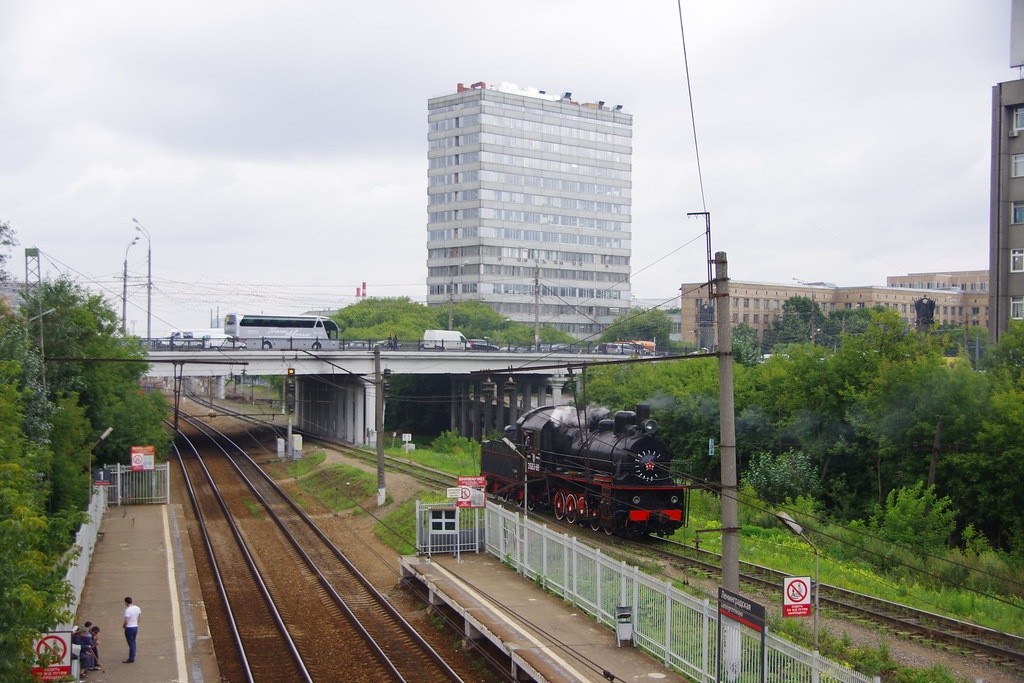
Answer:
[{"left": 480, "top": 360, "right": 690, "bottom": 541}]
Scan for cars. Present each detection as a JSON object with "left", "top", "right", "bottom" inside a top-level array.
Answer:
[
  {"left": 591, "top": 342, "right": 637, "bottom": 355},
  {"left": 466, "top": 338, "right": 498, "bottom": 351},
  {"left": 532, "top": 343, "right": 577, "bottom": 354}
]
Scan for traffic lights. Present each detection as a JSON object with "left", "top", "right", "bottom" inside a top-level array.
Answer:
[{"left": 288, "top": 368, "right": 296, "bottom": 395}]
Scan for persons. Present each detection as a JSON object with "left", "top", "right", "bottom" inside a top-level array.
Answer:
[
  {"left": 394, "top": 335, "right": 397, "bottom": 348},
  {"left": 122, "top": 596, "right": 141, "bottom": 663},
  {"left": 72, "top": 622, "right": 100, "bottom": 678},
  {"left": 386, "top": 335, "right": 392, "bottom": 349}
]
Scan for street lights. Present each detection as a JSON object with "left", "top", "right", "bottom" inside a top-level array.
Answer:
[
  {"left": 792, "top": 276, "right": 816, "bottom": 347},
  {"left": 121, "top": 218, "right": 152, "bottom": 352},
  {"left": 774, "top": 510, "right": 822, "bottom": 683},
  {"left": 497, "top": 318, "right": 510, "bottom": 350},
  {"left": 501, "top": 436, "right": 528, "bottom": 578},
  {"left": 88, "top": 426, "right": 114, "bottom": 503},
  {"left": 449, "top": 261, "right": 471, "bottom": 330}
]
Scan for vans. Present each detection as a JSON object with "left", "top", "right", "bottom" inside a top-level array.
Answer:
[
  {"left": 759, "top": 353, "right": 790, "bottom": 364},
  {"left": 202, "top": 334, "right": 247, "bottom": 350},
  {"left": 423, "top": 329, "right": 473, "bottom": 352}
]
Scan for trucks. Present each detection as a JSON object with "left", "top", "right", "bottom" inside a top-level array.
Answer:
[{"left": 162, "top": 328, "right": 225, "bottom": 347}]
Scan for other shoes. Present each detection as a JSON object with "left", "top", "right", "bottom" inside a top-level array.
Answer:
[
  {"left": 88, "top": 667, "right": 101, "bottom": 670},
  {"left": 95, "top": 663, "right": 104, "bottom": 666},
  {"left": 80, "top": 673, "right": 88, "bottom": 679},
  {"left": 122, "top": 659, "right": 134, "bottom": 663}
]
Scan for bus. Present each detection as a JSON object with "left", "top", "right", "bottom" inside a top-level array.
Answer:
[{"left": 223, "top": 312, "right": 342, "bottom": 352}]
